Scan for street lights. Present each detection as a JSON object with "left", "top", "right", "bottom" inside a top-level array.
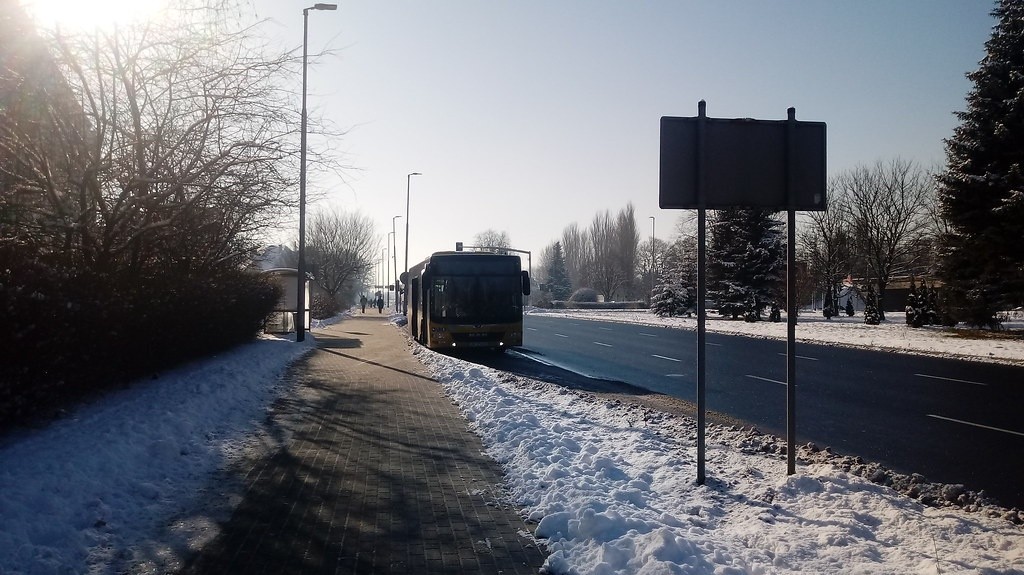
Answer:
[
  {"left": 372, "top": 215, "right": 403, "bottom": 310},
  {"left": 648, "top": 216, "right": 656, "bottom": 305},
  {"left": 295, "top": 3, "right": 338, "bottom": 342},
  {"left": 403, "top": 171, "right": 424, "bottom": 316}
]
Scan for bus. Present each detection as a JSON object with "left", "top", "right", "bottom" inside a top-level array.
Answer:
[{"left": 406, "top": 249, "right": 532, "bottom": 355}]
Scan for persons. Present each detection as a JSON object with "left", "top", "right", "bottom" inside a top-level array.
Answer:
[{"left": 360, "top": 296, "right": 384, "bottom": 314}]
